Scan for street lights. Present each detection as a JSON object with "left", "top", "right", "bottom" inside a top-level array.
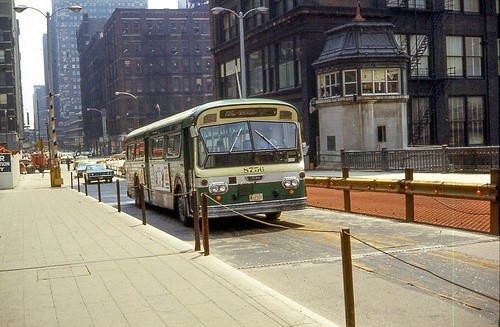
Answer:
[
  {"left": 211, "top": 6, "right": 270, "bottom": 98},
  {"left": 86, "top": 108, "right": 107, "bottom": 143},
  {"left": 14, "top": 4, "right": 83, "bottom": 188},
  {"left": 115, "top": 91, "right": 140, "bottom": 128},
  {"left": 36, "top": 94, "right": 61, "bottom": 151}
]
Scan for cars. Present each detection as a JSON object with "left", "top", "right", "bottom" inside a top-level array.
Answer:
[{"left": 0, "top": 145, "right": 126, "bottom": 185}]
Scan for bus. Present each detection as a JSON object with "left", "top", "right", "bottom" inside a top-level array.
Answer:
[{"left": 124, "top": 97, "right": 309, "bottom": 228}]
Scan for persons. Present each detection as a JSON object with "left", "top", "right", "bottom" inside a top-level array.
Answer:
[
  {"left": 66, "top": 156, "right": 71, "bottom": 171},
  {"left": 256, "top": 127, "right": 278, "bottom": 149}
]
